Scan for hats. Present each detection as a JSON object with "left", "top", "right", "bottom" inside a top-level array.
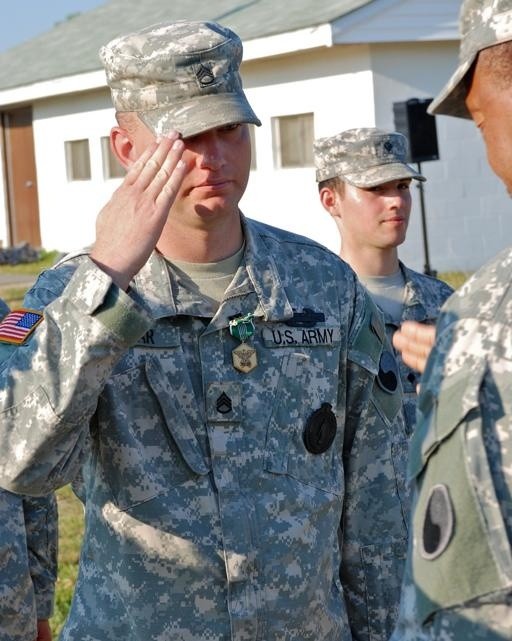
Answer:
[
  {"left": 424, "top": 0, "right": 512, "bottom": 120},
  {"left": 308, "top": 124, "right": 429, "bottom": 191},
  {"left": 96, "top": 17, "right": 263, "bottom": 147}
]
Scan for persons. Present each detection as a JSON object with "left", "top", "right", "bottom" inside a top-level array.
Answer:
[
  {"left": 1, "top": 301, "right": 62, "bottom": 641},
  {"left": 386, "top": 1, "right": 512, "bottom": 641},
  {"left": 290, "top": 123, "right": 456, "bottom": 641},
  {"left": 1, "top": 17, "right": 423, "bottom": 641}
]
